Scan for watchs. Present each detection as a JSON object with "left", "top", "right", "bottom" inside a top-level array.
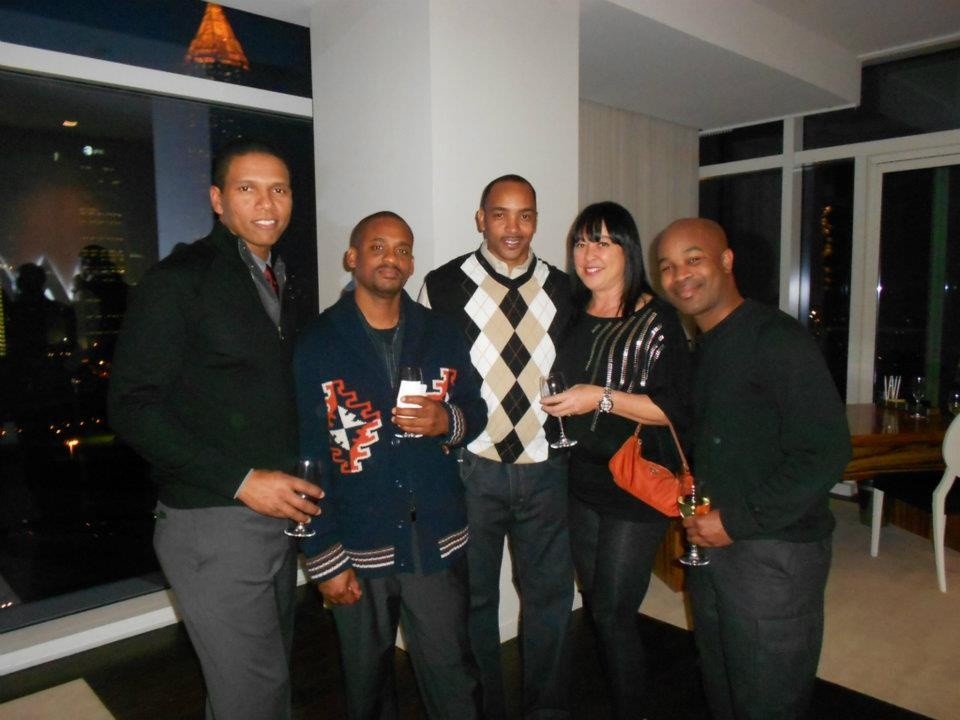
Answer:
[{"left": 596, "top": 385, "right": 613, "bottom": 413}]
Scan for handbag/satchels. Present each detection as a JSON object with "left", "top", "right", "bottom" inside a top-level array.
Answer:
[{"left": 607, "top": 392, "right": 694, "bottom": 518}]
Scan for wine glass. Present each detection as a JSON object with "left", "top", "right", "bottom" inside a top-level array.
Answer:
[
  {"left": 674, "top": 476, "right": 712, "bottom": 569},
  {"left": 393, "top": 363, "right": 427, "bottom": 439},
  {"left": 912, "top": 374, "right": 928, "bottom": 417},
  {"left": 539, "top": 370, "right": 577, "bottom": 451},
  {"left": 283, "top": 455, "right": 321, "bottom": 539}
]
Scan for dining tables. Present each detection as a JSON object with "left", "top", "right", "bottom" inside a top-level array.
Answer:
[{"left": 846, "top": 399, "right": 957, "bottom": 482}]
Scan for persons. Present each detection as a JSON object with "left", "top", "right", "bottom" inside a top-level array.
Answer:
[
  {"left": 417, "top": 174, "right": 591, "bottom": 720},
  {"left": 5, "top": 266, "right": 74, "bottom": 387},
  {"left": 104, "top": 138, "right": 325, "bottom": 720},
  {"left": 69, "top": 245, "right": 131, "bottom": 370},
  {"left": 539, "top": 196, "right": 687, "bottom": 720},
  {"left": 651, "top": 218, "right": 854, "bottom": 720},
  {"left": 291, "top": 209, "right": 484, "bottom": 720}
]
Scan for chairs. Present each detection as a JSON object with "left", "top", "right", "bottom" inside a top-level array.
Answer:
[{"left": 870, "top": 410, "right": 959, "bottom": 593}]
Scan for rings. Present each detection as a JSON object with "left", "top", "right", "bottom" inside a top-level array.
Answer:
[{"left": 566, "top": 411, "right": 572, "bottom": 418}]
